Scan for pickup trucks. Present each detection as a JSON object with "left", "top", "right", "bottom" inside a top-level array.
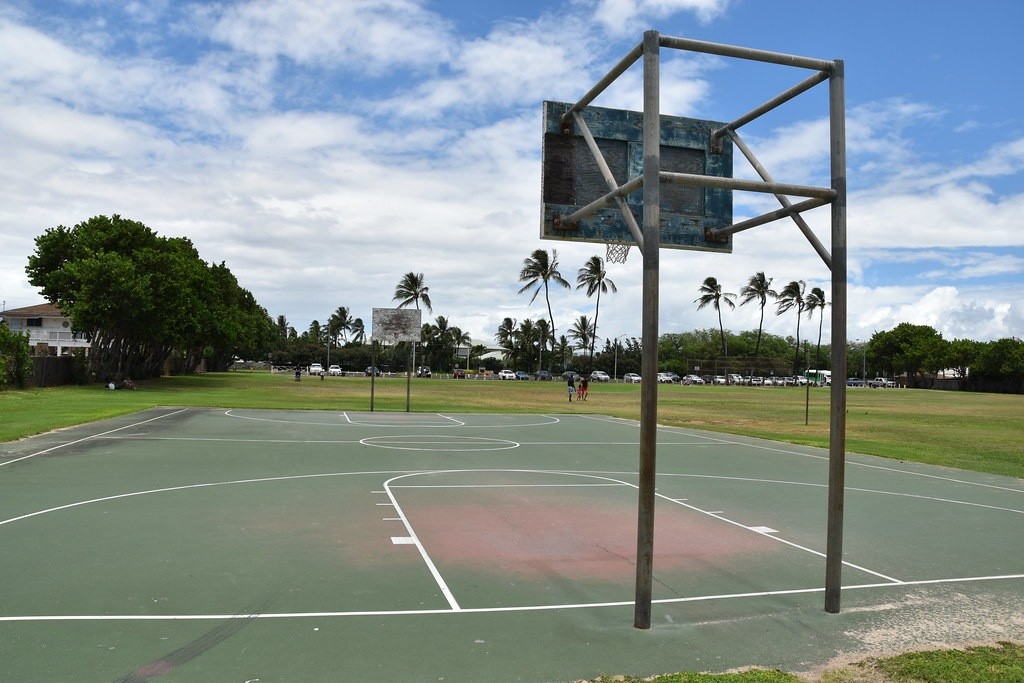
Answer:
[
  {"left": 846, "top": 377, "right": 864, "bottom": 387},
  {"left": 866, "top": 377, "right": 897, "bottom": 388}
]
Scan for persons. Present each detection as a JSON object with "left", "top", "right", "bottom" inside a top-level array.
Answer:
[
  {"left": 109, "top": 377, "right": 136, "bottom": 391},
  {"left": 568, "top": 375, "right": 576, "bottom": 402},
  {"left": 320, "top": 368, "right": 324, "bottom": 380},
  {"left": 295, "top": 364, "right": 301, "bottom": 381},
  {"left": 576, "top": 376, "right": 588, "bottom": 401}
]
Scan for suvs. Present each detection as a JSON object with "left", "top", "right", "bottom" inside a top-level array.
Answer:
[
  {"left": 416, "top": 366, "right": 431, "bottom": 378},
  {"left": 308, "top": 363, "right": 323, "bottom": 375}
]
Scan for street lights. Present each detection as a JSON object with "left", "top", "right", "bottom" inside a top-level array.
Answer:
[{"left": 856, "top": 338, "right": 867, "bottom": 388}]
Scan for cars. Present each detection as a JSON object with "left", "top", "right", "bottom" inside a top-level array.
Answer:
[
  {"left": 590, "top": 371, "right": 610, "bottom": 382},
  {"left": 561, "top": 371, "right": 580, "bottom": 382},
  {"left": 497, "top": 370, "right": 516, "bottom": 380},
  {"left": 364, "top": 366, "right": 380, "bottom": 377},
  {"left": 329, "top": 365, "right": 342, "bottom": 376},
  {"left": 623, "top": 372, "right": 642, "bottom": 383},
  {"left": 534, "top": 371, "right": 552, "bottom": 380},
  {"left": 682, "top": 375, "right": 706, "bottom": 385},
  {"left": 454, "top": 369, "right": 466, "bottom": 379},
  {"left": 713, "top": 375, "right": 726, "bottom": 385},
  {"left": 667, "top": 372, "right": 680, "bottom": 382},
  {"left": 700, "top": 375, "right": 713, "bottom": 384},
  {"left": 726, "top": 373, "right": 814, "bottom": 386},
  {"left": 657, "top": 373, "right": 673, "bottom": 384},
  {"left": 515, "top": 371, "right": 529, "bottom": 380}
]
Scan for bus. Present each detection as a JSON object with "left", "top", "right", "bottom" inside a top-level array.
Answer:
[{"left": 802, "top": 370, "right": 831, "bottom": 387}]
